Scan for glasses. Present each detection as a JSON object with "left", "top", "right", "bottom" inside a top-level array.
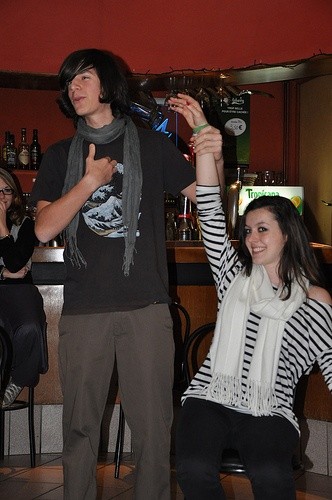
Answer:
[{"left": 0, "top": 188, "right": 13, "bottom": 195}]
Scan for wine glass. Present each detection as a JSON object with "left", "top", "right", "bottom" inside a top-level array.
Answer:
[{"left": 163, "top": 74, "right": 230, "bottom": 112}]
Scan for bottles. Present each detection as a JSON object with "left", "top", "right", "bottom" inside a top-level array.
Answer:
[
  {"left": 163, "top": 191, "right": 203, "bottom": 242},
  {"left": 17, "top": 128, "right": 30, "bottom": 169},
  {"left": 29, "top": 129, "right": 41, "bottom": 171},
  {"left": 1, "top": 132, "right": 17, "bottom": 169}
]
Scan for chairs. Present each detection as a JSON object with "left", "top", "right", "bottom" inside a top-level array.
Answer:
[{"left": 114, "top": 302, "right": 310, "bottom": 480}]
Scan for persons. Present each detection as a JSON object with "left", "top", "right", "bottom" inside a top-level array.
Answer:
[
  {"left": 168, "top": 93, "right": 332, "bottom": 500},
  {"left": 0, "top": 168, "right": 40, "bottom": 406},
  {"left": 28, "top": 49, "right": 225, "bottom": 500}
]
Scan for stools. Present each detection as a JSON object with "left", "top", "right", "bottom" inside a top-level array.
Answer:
[{"left": 1, "top": 386, "right": 47, "bottom": 469}]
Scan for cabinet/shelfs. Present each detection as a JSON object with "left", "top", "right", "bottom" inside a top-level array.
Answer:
[{"left": 0, "top": 55, "right": 332, "bottom": 260}]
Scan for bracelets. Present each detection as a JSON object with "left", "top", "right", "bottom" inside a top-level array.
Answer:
[
  {"left": 193, "top": 123, "right": 209, "bottom": 134},
  {"left": 0, "top": 234, "right": 10, "bottom": 240}
]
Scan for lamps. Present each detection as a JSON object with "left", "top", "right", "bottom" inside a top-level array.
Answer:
[{"left": 219, "top": 71, "right": 231, "bottom": 78}]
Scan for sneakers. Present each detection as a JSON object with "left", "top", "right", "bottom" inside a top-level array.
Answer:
[{"left": 2, "top": 381, "right": 24, "bottom": 408}]
